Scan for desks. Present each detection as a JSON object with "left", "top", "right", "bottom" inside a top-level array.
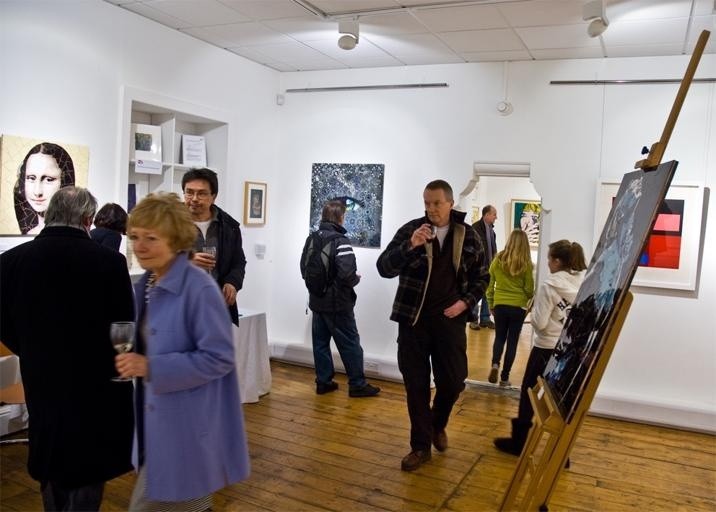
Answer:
[{"left": 232, "top": 310, "right": 273, "bottom": 404}]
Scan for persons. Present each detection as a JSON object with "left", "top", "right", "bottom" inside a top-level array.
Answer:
[
  {"left": 375, "top": 180, "right": 490, "bottom": 471},
  {"left": 13, "top": 141, "right": 75, "bottom": 235},
  {"left": 112, "top": 192, "right": 250, "bottom": 512},
  {"left": 181, "top": 167, "right": 246, "bottom": 330},
  {"left": 299, "top": 198, "right": 380, "bottom": 397},
  {"left": 0, "top": 186, "right": 138, "bottom": 512},
  {"left": 468, "top": 205, "right": 495, "bottom": 330},
  {"left": 494, "top": 238, "right": 589, "bottom": 468},
  {"left": 488, "top": 230, "right": 534, "bottom": 387},
  {"left": 89, "top": 204, "right": 132, "bottom": 273}
]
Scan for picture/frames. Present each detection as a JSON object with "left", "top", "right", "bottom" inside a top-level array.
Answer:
[
  {"left": 244, "top": 182, "right": 267, "bottom": 225},
  {"left": 509, "top": 198, "right": 542, "bottom": 249},
  {"left": 595, "top": 180, "right": 705, "bottom": 292}
]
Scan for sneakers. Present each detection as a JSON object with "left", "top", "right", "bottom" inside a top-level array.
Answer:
[
  {"left": 431, "top": 424, "right": 448, "bottom": 451},
  {"left": 348, "top": 383, "right": 381, "bottom": 397},
  {"left": 401, "top": 445, "right": 431, "bottom": 472},
  {"left": 469, "top": 321, "right": 480, "bottom": 330},
  {"left": 315, "top": 377, "right": 339, "bottom": 395},
  {"left": 478, "top": 319, "right": 496, "bottom": 328},
  {"left": 488, "top": 368, "right": 498, "bottom": 382},
  {"left": 500, "top": 379, "right": 512, "bottom": 386}
]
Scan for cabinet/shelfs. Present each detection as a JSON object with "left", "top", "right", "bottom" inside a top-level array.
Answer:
[{"left": 120, "top": 84, "right": 234, "bottom": 214}]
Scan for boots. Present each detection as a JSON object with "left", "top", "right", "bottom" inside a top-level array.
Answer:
[{"left": 493, "top": 417, "right": 532, "bottom": 457}]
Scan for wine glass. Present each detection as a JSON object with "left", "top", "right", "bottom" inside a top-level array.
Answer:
[
  {"left": 109, "top": 320, "right": 138, "bottom": 384},
  {"left": 418, "top": 225, "right": 438, "bottom": 258},
  {"left": 201, "top": 245, "right": 218, "bottom": 277}
]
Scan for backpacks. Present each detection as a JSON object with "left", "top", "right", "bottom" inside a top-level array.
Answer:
[{"left": 304, "top": 232, "right": 337, "bottom": 296}]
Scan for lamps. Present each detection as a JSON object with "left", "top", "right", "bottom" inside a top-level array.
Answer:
[
  {"left": 339, "top": 17, "right": 360, "bottom": 49},
  {"left": 584, "top": 0, "right": 609, "bottom": 36}
]
